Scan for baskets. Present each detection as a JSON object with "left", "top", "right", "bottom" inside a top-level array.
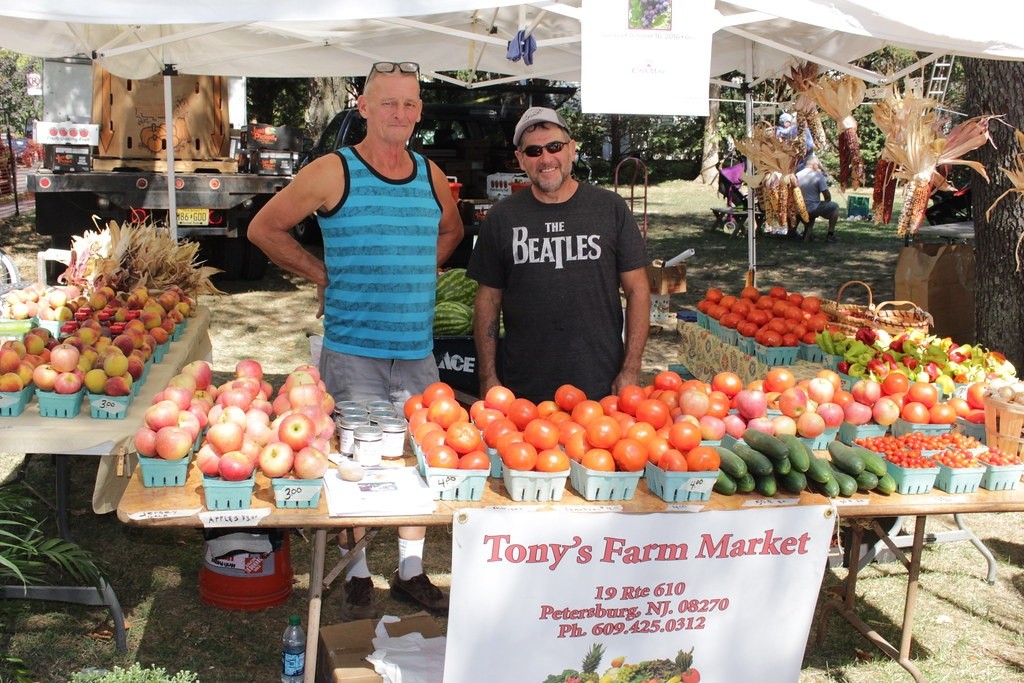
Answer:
[{"left": 0, "top": 280, "right": 1024, "bottom": 508}]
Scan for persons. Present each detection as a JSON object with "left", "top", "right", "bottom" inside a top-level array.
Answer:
[
  {"left": 775, "top": 113, "right": 840, "bottom": 242},
  {"left": 465, "top": 109, "right": 652, "bottom": 405},
  {"left": 247, "top": 66, "right": 464, "bottom": 618}
]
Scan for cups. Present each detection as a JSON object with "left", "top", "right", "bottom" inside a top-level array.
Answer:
[{"left": 738, "top": 206, "right": 743, "bottom": 213}]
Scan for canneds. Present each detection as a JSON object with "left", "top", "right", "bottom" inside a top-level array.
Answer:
[{"left": 334, "top": 400, "right": 408, "bottom": 468}]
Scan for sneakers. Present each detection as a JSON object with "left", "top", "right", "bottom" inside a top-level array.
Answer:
[
  {"left": 340, "top": 575, "right": 376, "bottom": 622},
  {"left": 391, "top": 574, "right": 448, "bottom": 616}
]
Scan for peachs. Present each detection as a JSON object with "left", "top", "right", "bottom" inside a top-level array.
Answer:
[
  {"left": 0, "top": 286, "right": 195, "bottom": 363},
  {"left": 0, "top": 348, "right": 35, "bottom": 392},
  {"left": 60, "top": 319, "right": 158, "bottom": 395}
]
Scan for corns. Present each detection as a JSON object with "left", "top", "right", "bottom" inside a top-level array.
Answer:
[
  {"left": 796, "top": 109, "right": 828, "bottom": 153},
  {"left": 762, "top": 183, "right": 809, "bottom": 229},
  {"left": 838, "top": 127, "right": 932, "bottom": 238}
]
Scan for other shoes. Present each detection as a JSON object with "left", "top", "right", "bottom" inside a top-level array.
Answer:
[{"left": 825, "top": 235, "right": 840, "bottom": 243}]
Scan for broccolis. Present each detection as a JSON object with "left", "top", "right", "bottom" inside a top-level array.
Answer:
[{"left": 542, "top": 669, "right": 579, "bottom": 683}]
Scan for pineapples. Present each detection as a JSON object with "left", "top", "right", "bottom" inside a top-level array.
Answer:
[{"left": 581, "top": 643, "right": 607, "bottom": 683}]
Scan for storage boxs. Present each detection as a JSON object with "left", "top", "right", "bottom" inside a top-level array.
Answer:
[
  {"left": 238, "top": 124, "right": 305, "bottom": 177},
  {"left": 319, "top": 611, "right": 446, "bottom": 683},
  {"left": 648, "top": 265, "right": 689, "bottom": 295},
  {"left": 485, "top": 172, "right": 531, "bottom": 199},
  {"left": 1, "top": 285, "right": 328, "bottom": 509},
  {"left": 32, "top": 121, "right": 101, "bottom": 171},
  {"left": 445, "top": 176, "right": 463, "bottom": 204},
  {"left": 405, "top": 294, "right": 1024, "bottom": 502}
]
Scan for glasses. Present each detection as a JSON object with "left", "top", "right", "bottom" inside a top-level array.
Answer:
[
  {"left": 364, "top": 62, "right": 421, "bottom": 92},
  {"left": 518, "top": 141, "right": 571, "bottom": 158}
]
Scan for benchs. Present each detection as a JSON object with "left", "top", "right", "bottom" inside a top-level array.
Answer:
[{"left": 710, "top": 207, "right": 813, "bottom": 243}]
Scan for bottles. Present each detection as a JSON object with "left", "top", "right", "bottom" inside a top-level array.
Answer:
[
  {"left": 79, "top": 308, "right": 93, "bottom": 319},
  {"left": 103, "top": 309, "right": 115, "bottom": 326},
  {"left": 65, "top": 320, "right": 78, "bottom": 333},
  {"left": 128, "top": 310, "right": 140, "bottom": 320},
  {"left": 280, "top": 615, "right": 306, "bottom": 683},
  {"left": 110, "top": 326, "right": 123, "bottom": 342},
  {"left": 58, "top": 326, "right": 74, "bottom": 344},
  {"left": 98, "top": 314, "right": 111, "bottom": 338},
  {"left": 125, "top": 313, "right": 136, "bottom": 325},
  {"left": 113, "top": 322, "right": 127, "bottom": 331},
  {"left": 74, "top": 313, "right": 89, "bottom": 329}
]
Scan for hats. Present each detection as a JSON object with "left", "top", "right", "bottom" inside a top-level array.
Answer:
[
  {"left": 779, "top": 112, "right": 792, "bottom": 124},
  {"left": 512, "top": 107, "right": 570, "bottom": 145}
]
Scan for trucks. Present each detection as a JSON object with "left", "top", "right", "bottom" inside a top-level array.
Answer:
[{"left": 28, "top": 59, "right": 313, "bottom": 285}]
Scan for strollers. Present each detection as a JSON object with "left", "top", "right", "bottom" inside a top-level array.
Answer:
[{"left": 925, "top": 180, "right": 973, "bottom": 226}]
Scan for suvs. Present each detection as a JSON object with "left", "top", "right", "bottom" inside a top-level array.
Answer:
[{"left": 296, "top": 87, "right": 576, "bottom": 243}]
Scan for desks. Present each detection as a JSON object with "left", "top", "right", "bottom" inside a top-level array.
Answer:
[
  {"left": 680, "top": 311, "right": 996, "bottom": 589},
  {"left": 1, "top": 303, "right": 212, "bottom": 658},
  {"left": 112, "top": 441, "right": 1024, "bottom": 683}
]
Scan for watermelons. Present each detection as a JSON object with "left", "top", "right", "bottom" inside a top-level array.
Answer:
[{"left": 434, "top": 267, "right": 504, "bottom": 338}]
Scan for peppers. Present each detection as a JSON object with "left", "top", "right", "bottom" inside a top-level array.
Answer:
[
  {"left": 681, "top": 667, "right": 701, "bottom": 683},
  {"left": 813, "top": 323, "right": 1016, "bottom": 401}
]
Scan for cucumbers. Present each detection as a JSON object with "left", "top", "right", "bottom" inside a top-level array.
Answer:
[{"left": 712, "top": 430, "right": 896, "bottom": 499}]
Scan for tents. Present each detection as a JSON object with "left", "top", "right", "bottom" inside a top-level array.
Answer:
[{"left": 0, "top": 0, "right": 1024, "bottom": 271}]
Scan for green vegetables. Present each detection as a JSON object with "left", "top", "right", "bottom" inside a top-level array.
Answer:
[{"left": 629, "top": 647, "right": 695, "bottom": 683}]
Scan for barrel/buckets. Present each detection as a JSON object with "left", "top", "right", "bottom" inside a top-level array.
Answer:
[
  {"left": 509, "top": 182, "right": 532, "bottom": 194},
  {"left": 448, "top": 182, "right": 462, "bottom": 204},
  {"left": 199, "top": 528, "right": 293, "bottom": 610}
]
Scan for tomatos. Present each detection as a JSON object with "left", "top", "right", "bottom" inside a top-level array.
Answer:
[
  {"left": 698, "top": 286, "right": 842, "bottom": 348},
  {"left": 855, "top": 432, "right": 1020, "bottom": 469},
  {"left": 746, "top": 368, "right": 856, "bottom": 409},
  {"left": 404, "top": 372, "right": 743, "bottom": 474},
  {"left": 878, "top": 372, "right": 991, "bottom": 424}
]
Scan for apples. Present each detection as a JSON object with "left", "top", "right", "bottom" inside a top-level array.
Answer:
[
  {"left": 31, "top": 343, "right": 84, "bottom": 394},
  {"left": 677, "top": 377, "right": 898, "bottom": 438},
  {"left": 134, "top": 361, "right": 338, "bottom": 481}
]
